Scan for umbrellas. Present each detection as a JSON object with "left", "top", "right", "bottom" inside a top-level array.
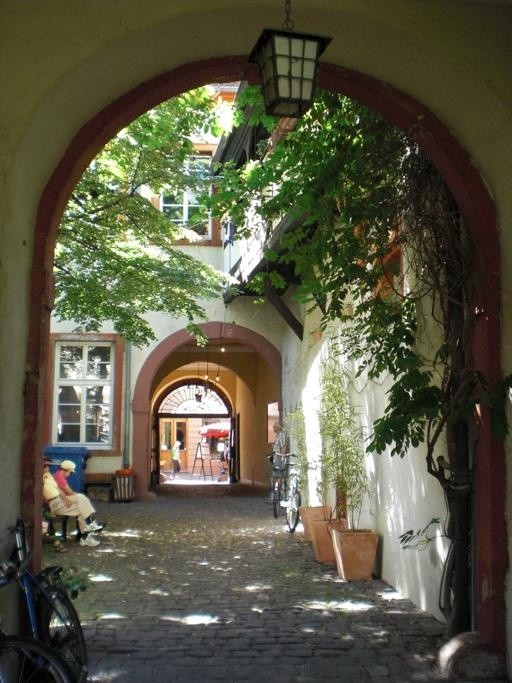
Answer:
[{"left": 202, "top": 422, "right": 233, "bottom": 482}]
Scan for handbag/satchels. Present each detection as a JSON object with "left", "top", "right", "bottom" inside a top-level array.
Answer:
[{"left": 268, "top": 455, "right": 274, "bottom": 463}]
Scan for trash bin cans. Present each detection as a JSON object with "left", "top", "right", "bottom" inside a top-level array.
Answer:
[{"left": 44, "top": 446, "right": 89, "bottom": 493}]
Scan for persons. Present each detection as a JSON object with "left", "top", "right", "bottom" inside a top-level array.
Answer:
[
  {"left": 42, "top": 459, "right": 104, "bottom": 546},
  {"left": 269, "top": 423, "right": 289, "bottom": 500},
  {"left": 53, "top": 460, "right": 96, "bottom": 540},
  {"left": 170, "top": 442, "right": 182, "bottom": 476}
]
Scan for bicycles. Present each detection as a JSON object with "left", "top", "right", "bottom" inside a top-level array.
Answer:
[
  {"left": 0, "top": 520, "right": 90, "bottom": 682},
  {"left": 266, "top": 450, "right": 304, "bottom": 532}
]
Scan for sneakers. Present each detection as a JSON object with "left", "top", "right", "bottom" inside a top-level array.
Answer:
[{"left": 75, "top": 520, "right": 108, "bottom": 548}]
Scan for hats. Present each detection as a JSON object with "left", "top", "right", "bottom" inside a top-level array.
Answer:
[
  {"left": 60, "top": 459, "right": 77, "bottom": 473},
  {"left": 43, "top": 455, "right": 52, "bottom": 468}
]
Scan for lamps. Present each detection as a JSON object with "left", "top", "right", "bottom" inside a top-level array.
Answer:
[{"left": 241, "top": 0, "right": 335, "bottom": 119}]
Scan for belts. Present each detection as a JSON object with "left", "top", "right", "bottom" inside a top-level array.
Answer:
[{"left": 47, "top": 494, "right": 62, "bottom": 503}]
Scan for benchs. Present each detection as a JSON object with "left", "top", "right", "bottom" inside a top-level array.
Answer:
[{"left": 40, "top": 504, "right": 70, "bottom": 538}]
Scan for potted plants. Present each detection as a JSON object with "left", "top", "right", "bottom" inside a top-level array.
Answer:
[{"left": 280, "top": 358, "right": 381, "bottom": 585}]
